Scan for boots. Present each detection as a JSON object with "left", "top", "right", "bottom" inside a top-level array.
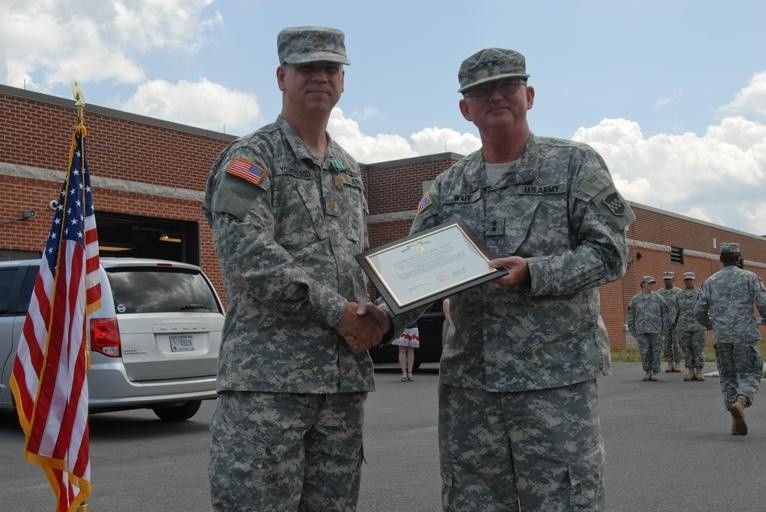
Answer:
[
  {"left": 651, "top": 371, "right": 659, "bottom": 381},
  {"left": 674, "top": 361, "right": 682, "bottom": 373},
  {"left": 730, "top": 393, "right": 749, "bottom": 435},
  {"left": 684, "top": 367, "right": 695, "bottom": 381},
  {"left": 696, "top": 368, "right": 705, "bottom": 381},
  {"left": 642, "top": 370, "right": 651, "bottom": 381},
  {"left": 665, "top": 361, "right": 674, "bottom": 373}
]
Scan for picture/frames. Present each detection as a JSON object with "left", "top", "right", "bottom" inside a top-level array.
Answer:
[{"left": 353, "top": 217, "right": 509, "bottom": 316}]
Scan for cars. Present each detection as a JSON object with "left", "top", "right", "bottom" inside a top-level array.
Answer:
[{"left": 369, "top": 303, "right": 456, "bottom": 372}]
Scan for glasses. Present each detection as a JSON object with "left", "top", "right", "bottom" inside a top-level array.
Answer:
[{"left": 463, "top": 78, "right": 527, "bottom": 101}]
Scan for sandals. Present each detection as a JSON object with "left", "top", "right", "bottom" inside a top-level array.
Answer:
[
  {"left": 408, "top": 371, "right": 415, "bottom": 382},
  {"left": 401, "top": 371, "right": 408, "bottom": 382}
]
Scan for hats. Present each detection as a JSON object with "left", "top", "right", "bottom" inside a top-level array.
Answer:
[
  {"left": 683, "top": 271, "right": 697, "bottom": 280},
  {"left": 276, "top": 25, "right": 352, "bottom": 66},
  {"left": 720, "top": 242, "right": 741, "bottom": 253},
  {"left": 662, "top": 272, "right": 675, "bottom": 279},
  {"left": 457, "top": 48, "right": 531, "bottom": 93},
  {"left": 640, "top": 276, "right": 656, "bottom": 285}
]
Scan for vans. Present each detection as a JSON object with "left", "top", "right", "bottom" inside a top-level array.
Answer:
[{"left": 0, "top": 252, "right": 224, "bottom": 424}]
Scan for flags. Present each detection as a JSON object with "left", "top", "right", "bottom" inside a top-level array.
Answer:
[{"left": 8, "top": 125, "right": 102, "bottom": 512}]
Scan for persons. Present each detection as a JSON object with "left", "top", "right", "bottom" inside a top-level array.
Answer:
[
  {"left": 657, "top": 271, "right": 683, "bottom": 372},
  {"left": 442, "top": 299, "right": 452, "bottom": 348},
  {"left": 346, "top": 48, "right": 637, "bottom": 512},
  {"left": 671, "top": 272, "right": 707, "bottom": 381},
  {"left": 391, "top": 322, "right": 419, "bottom": 382},
  {"left": 202, "top": 26, "right": 384, "bottom": 512},
  {"left": 694, "top": 242, "right": 766, "bottom": 435},
  {"left": 627, "top": 276, "right": 669, "bottom": 381}
]
